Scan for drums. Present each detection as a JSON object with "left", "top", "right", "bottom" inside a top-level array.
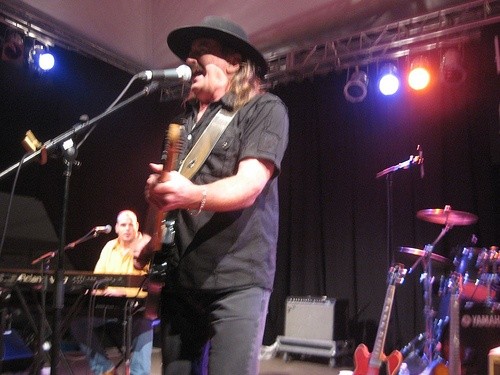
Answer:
[{"left": 450, "top": 247, "right": 500, "bottom": 301}]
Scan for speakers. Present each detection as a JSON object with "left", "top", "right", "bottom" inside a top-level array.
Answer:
[
  {"left": 284, "top": 295, "right": 347, "bottom": 340},
  {"left": 0, "top": 329, "right": 33, "bottom": 375},
  {"left": 0, "top": 192, "right": 59, "bottom": 254}
]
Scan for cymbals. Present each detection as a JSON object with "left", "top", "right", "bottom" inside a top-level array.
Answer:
[{"left": 416, "top": 209, "right": 478, "bottom": 225}]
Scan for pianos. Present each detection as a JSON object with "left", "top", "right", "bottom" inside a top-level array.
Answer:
[{"left": 0, "top": 269, "right": 146, "bottom": 375}]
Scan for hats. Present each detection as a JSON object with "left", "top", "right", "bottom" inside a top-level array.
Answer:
[{"left": 167, "top": 16, "right": 271, "bottom": 76}]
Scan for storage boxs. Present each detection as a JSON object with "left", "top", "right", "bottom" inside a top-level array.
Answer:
[{"left": 276, "top": 334, "right": 355, "bottom": 357}]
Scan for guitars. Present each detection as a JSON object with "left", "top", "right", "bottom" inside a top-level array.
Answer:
[
  {"left": 145, "top": 122, "right": 191, "bottom": 321},
  {"left": 353, "top": 262, "right": 407, "bottom": 375}
]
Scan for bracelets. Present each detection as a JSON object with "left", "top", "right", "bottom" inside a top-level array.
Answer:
[{"left": 187, "top": 184, "right": 206, "bottom": 216}]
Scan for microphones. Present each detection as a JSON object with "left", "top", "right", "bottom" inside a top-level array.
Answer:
[
  {"left": 94, "top": 225, "right": 111, "bottom": 234},
  {"left": 416, "top": 144, "right": 424, "bottom": 178},
  {"left": 137, "top": 64, "right": 192, "bottom": 81}
]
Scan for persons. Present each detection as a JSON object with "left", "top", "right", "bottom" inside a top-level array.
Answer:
[
  {"left": 146, "top": 15, "right": 289, "bottom": 375},
  {"left": 72, "top": 210, "right": 153, "bottom": 375}
]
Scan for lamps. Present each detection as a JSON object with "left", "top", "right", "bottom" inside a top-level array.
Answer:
[
  {"left": 1, "top": 27, "right": 26, "bottom": 64},
  {"left": 28, "top": 38, "right": 55, "bottom": 71},
  {"left": 407, "top": 50, "right": 430, "bottom": 91},
  {"left": 439, "top": 43, "right": 467, "bottom": 87},
  {"left": 375, "top": 57, "right": 400, "bottom": 97},
  {"left": 344, "top": 62, "right": 370, "bottom": 103}
]
